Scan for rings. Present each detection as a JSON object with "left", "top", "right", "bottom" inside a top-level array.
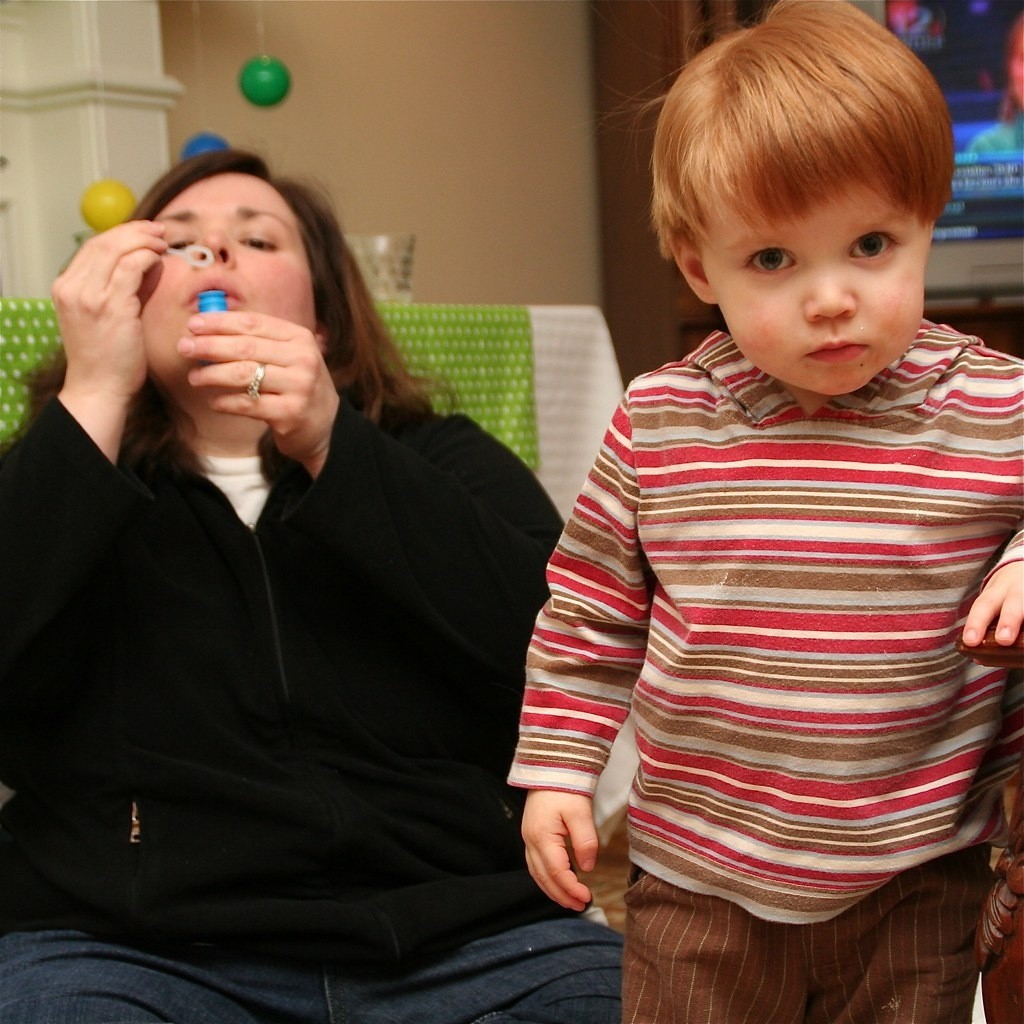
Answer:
[{"left": 247, "top": 360, "right": 264, "bottom": 401}]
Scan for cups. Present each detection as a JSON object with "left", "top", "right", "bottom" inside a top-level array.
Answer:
[{"left": 342, "top": 231, "right": 417, "bottom": 305}]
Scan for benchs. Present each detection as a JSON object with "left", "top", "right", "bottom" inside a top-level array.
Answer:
[{"left": 0, "top": 299, "right": 630, "bottom": 515}]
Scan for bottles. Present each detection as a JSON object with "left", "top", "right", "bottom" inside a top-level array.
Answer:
[{"left": 198, "top": 290, "right": 228, "bottom": 367}]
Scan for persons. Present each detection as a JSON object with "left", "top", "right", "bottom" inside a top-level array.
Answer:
[
  {"left": 509, "top": 0, "right": 1024, "bottom": 1024},
  {"left": 967, "top": 12, "right": 1024, "bottom": 156},
  {"left": 0, "top": 147, "right": 642, "bottom": 1024}
]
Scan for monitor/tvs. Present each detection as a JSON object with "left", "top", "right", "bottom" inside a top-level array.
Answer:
[{"left": 850, "top": 0, "right": 1024, "bottom": 297}]
